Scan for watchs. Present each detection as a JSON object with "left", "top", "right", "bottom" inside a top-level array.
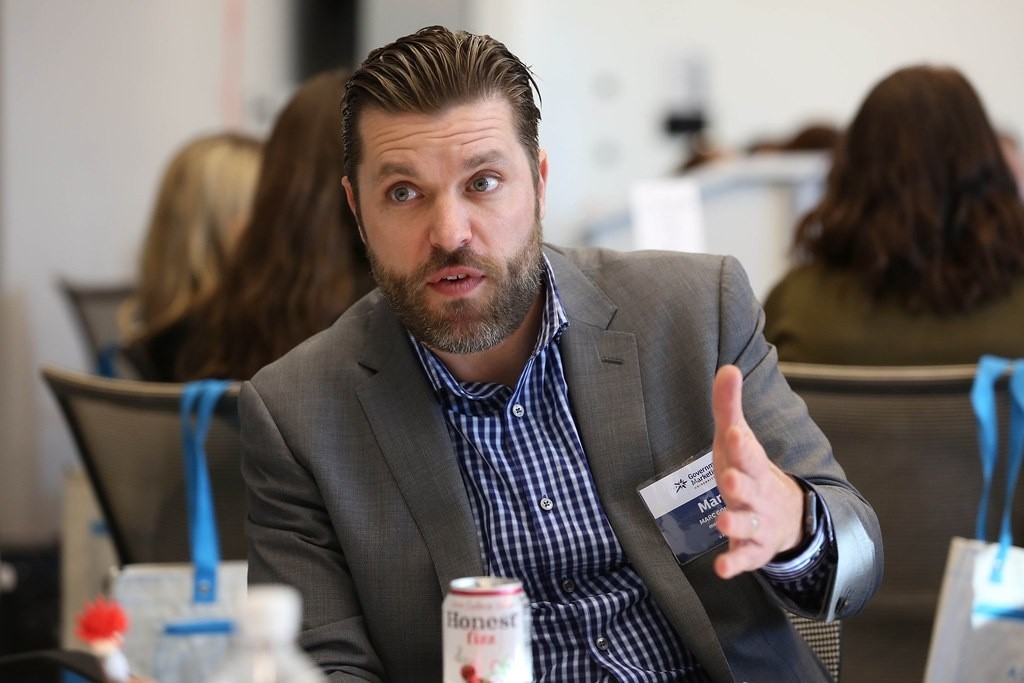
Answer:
[{"left": 802, "top": 487, "right": 818, "bottom": 538}]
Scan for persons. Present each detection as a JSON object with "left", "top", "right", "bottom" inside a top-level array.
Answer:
[
  {"left": 130, "top": 66, "right": 375, "bottom": 387},
  {"left": 120, "top": 136, "right": 262, "bottom": 345},
  {"left": 241, "top": 26, "right": 884, "bottom": 683},
  {"left": 760, "top": 64, "right": 1024, "bottom": 368}
]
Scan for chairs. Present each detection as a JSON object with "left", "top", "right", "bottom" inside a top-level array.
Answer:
[
  {"left": 776, "top": 362, "right": 1024, "bottom": 683},
  {"left": 36, "top": 365, "right": 250, "bottom": 565}
]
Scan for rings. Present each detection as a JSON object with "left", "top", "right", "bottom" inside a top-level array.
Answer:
[{"left": 752, "top": 515, "right": 760, "bottom": 534}]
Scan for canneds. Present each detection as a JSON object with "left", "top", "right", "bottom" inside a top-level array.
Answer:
[{"left": 441, "top": 575, "right": 534, "bottom": 683}]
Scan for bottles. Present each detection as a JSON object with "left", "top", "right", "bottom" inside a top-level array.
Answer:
[
  {"left": 205, "top": 585, "right": 327, "bottom": 683},
  {"left": 76, "top": 599, "right": 156, "bottom": 683}
]
